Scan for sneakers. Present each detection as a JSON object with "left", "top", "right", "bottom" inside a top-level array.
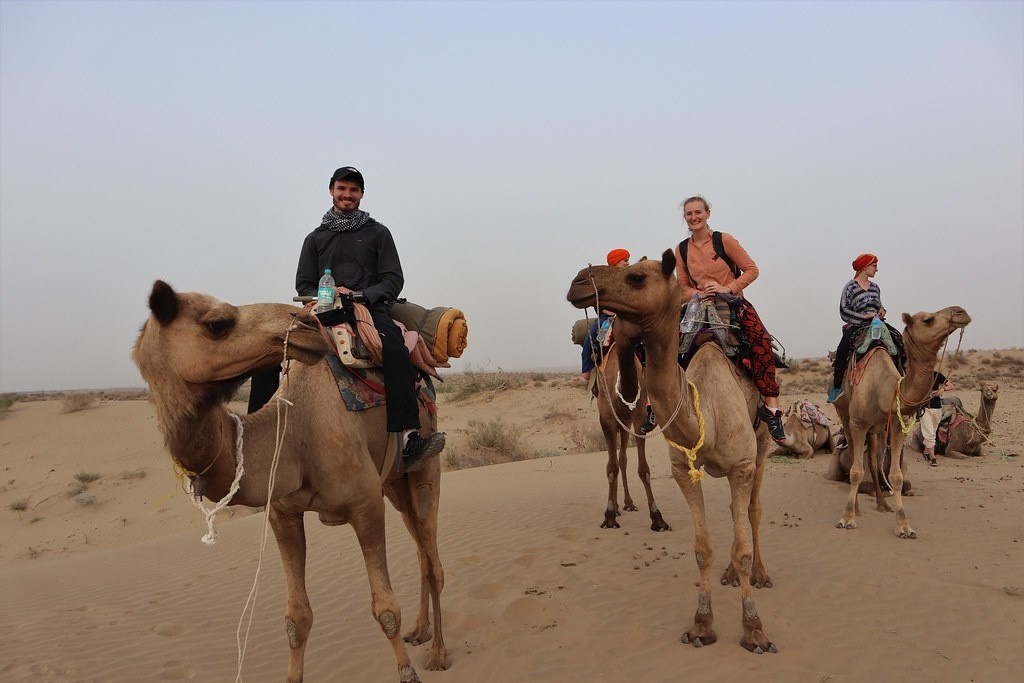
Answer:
[
  {"left": 402, "top": 431, "right": 446, "bottom": 473},
  {"left": 827, "top": 388, "right": 844, "bottom": 403},
  {"left": 568, "top": 376, "right": 590, "bottom": 390},
  {"left": 758, "top": 403, "right": 786, "bottom": 442},
  {"left": 640, "top": 405, "right": 658, "bottom": 433}
]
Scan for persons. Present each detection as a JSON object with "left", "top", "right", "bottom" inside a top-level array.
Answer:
[
  {"left": 825, "top": 253, "right": 905, "bottom": 403},
  {"left": 918, "top": 370, "right": 954, "bottom": 466},
  {"left": 639, "top": 195, "right": 786, "bottom": 442},
  {"left": 571, "top": 248, "right": 630, "bottom": 390},
  {"left": 246, "top": 165, "right": 445, "bottom": 473}
]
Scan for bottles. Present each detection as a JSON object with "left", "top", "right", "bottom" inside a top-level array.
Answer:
[
  {"left": 597, "top": 318, "right": 611, "bottom": 341},
  {"left": 680, "top": 294, "right": 699, "bottom": 332},
  {"left": 317, "top": 269, "right": 335, "bottom": 330},
  {"left": 871, "top": 313, "right": 881, "bottom": 340}
]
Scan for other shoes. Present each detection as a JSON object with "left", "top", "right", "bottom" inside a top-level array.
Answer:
[
  {"left": 923, "top": 452, "right": 930, "bottom": 461},
  {"left": 930, "top": 458, "right": 938, "bottom": 466}
]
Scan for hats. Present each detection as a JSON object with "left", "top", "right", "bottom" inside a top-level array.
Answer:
[
  {"left": 331, "top": 167, "right": 364, "bottom": 187},
  {"left": 852, "top": 254, "right": 878, "bottom": 271},
  {"left": 607, "top": 249, "right": 630, "bottom": 266}
]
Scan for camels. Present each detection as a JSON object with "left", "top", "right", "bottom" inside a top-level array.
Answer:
[
  {"left": 825, "top": 305, "right": 973, "bottom": 540},
  {"left": 767, "top": 399, "right": 839, "bottom": 460},
  {"left": 564, "top": 246, "right": 779, "bottom": 656},
  {"left": 129, "top": 278, "right": 452, "bottom": 683},
  {"left": 909, "top": 381, "right": 999, "bottom": 459}
]
